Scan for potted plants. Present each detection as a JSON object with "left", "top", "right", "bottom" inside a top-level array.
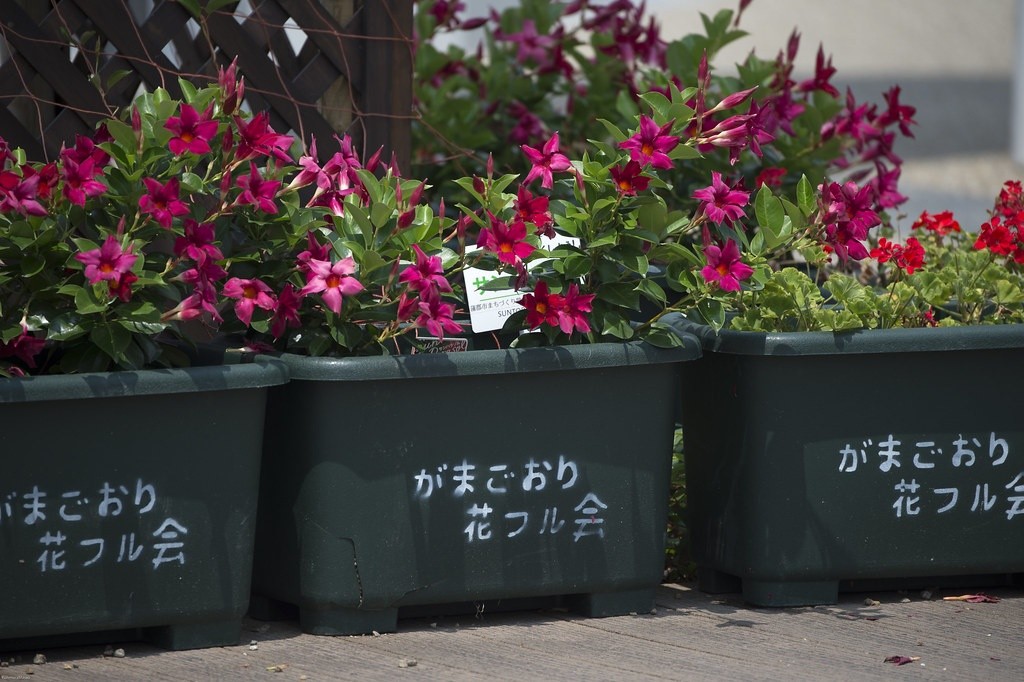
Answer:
[
  {"left": 662, "top": 282, "right": 1022, "bottom": 609},
  {"left": 262, "top": 313, "right": 698, "bottom": 629},
  {"left": 2, "top": 349, "right": 292, "bottom": 649}
]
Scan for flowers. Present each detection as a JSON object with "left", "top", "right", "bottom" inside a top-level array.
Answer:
[
  {"left": 0, "top": 62, "right": 290, "bottom": 373},
  {"left": 705, "top": 175, "right": 1022, "bottom": 336},
  {"left": 280, "top": 60, "right": 761, "bottom": 322}
]
